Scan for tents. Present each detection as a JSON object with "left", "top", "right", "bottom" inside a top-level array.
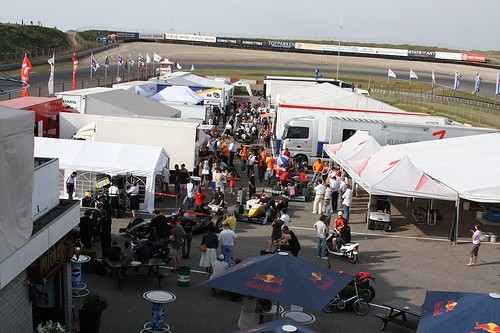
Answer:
[
  {"left": 149, "top": 85, "right": 205, "bottom": 106},
  {"left": 323, "top": 132, "right": 500, "bottom": 246},
  {"left": 33, "top": 89, "right": 209, "bottom": 212}
]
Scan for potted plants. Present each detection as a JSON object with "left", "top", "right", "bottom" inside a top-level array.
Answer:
[{"left": 79, "top": 291, "right": 110, "bottom": 333}]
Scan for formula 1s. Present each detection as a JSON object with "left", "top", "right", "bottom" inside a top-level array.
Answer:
[
  {"left": 234, "top": 189, "right": 290, "bottom": 225},
  {"left": 119, "top": 204, "right": 225, "bottom": 248}
]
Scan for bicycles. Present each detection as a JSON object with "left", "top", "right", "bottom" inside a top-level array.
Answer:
[{"left": 322, "top": 275, "right": 370, "bottom": 316}]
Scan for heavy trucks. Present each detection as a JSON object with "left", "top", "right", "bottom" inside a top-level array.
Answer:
[
  {"left": 265, "top": 75, "right": 369, "bottom": 98},
  {"left": 140, "top": 84, "right": 226, "bottom": 115},
  {"left": 69, "top": 118, "right": 200, "bottom": 176},
  {"left": 279, "top": 115, "right": 500, "bottom": 173}
]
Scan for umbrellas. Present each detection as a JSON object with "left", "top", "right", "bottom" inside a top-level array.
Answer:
[
  {"left": 416, "top": 291, "right": 500, "bottom": 333},
  {"left": 275, "top": 156, "right": 289, "bottom": 165},
  {"left": 232, "top": 317, "right": 321, "bottom": 333},
  {"left": 199, "top": 252, "right": 354, "bottom": 320}
]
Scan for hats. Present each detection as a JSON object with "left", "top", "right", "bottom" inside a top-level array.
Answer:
[
  {"left": 338, "top": 211, "right": 343, "bottom": 216},
  {"left": 219, "top": 254, "right": 224, "bottom": 260}
]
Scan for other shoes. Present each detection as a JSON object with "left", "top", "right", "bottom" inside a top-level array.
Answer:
[
  {"left": 321, "top": 256, "right": 329, "bottom": 260},
  {"left": 465, "top": 262, "right": 475, "bottom": 267},
  {"left": 183, "top": 255, "right": 189, "bottom": 259}
]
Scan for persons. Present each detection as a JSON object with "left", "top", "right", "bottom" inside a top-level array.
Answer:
[
  {"left": 465, "top": 225, "right": 483, "bottom": 267},
  {"left": 315, "top": 67, "right": 319, "bottom": 79},
  {"left": 66, "top": 172, "right": 77, "bottom": 199},
  {"left": 84, "top": 100, "right": 354, "bottom": 279},
  {"left": 237, "top": 295, "right": 273, "bottom": 330},
  {"left": 352, "top": 83, "right": 354, "bottom": 92}
]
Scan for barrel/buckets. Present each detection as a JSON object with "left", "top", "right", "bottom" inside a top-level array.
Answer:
[
  {"left": 476, "top": 212, "right": 483, "bottom": 218},
  {"left": 463, "top": 202, "right": 470, "bottom": 210},
  {"left": 481, "top": 232, "right": 497, "bottom": 243},
  {"left": 177, "top": 266, "right": 190, "bottom": 288}
]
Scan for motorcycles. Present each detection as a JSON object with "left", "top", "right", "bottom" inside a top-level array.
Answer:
[
  {"left": 324, "top": 229, "right": 359, "bottom": 263},
  {"left": 327, "top": 258, "right": 376, "bottom": 302}
]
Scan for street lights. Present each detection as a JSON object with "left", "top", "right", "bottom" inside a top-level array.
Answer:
[{"left": 336, "top": 25, "right": 342, "bottom": 80}]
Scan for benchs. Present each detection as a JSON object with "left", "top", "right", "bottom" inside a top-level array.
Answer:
[
  {"left": 375, "top": 312, "right": 417, "bottom": 331},
  {"left": 114, "top": 272, "right": 171, "bottom": 280}
]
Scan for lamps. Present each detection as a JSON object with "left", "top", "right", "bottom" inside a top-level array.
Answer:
[
  {"left": 28, "top": 274, "right": 47, "bottom": 303},
  {"left": 64, "top": 240, "right": 81, "bottom": 263}
]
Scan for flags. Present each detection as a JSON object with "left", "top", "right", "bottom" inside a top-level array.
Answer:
[
  {"left": 147, "top": 54, "right": 151, "bottom": 63},
  {"left": 118, "top": 54, "right": 123, "bottom": 66},
  {"left": 47, "top": 56, "right": 54, "bottom": 95},
  {"left": 176, "top": 61, "right": 183, "bottom": 70},
  {"left": 129, "top": 56, "right": 135, "bottom": 67},
  {"left": 139, "top": 55, "right": 145, "bottom": 64},
  {"left": 21, "top": 56, "right": 32, "bottom": 97},
  {"left": 388, "top": 68, "right": 500, "bottom": 95},
  {"left": 72, "top": 55, "right": 79, "bottom": 89},
  {"left": 92, "top": 57, "right": 100, "bottom": 72},
  {"left": 105, "top": 54, "right": 110, "bottom": 69},
  {"left": 154, "top": 53, "right": 162, "bottom": 63}
]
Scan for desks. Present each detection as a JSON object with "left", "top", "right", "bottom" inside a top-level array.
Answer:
[
  {"left": 255, "top": 302, "right": 284, "bottom": 323},
  {"left": 113, "top": 257, "right": 166, "bottom": 289},
  {"left": 281, "top": 310, "right": 316, "bottom": 325},
  {"left": 382, "top": 299, "right": 421, "bottom": 330}
]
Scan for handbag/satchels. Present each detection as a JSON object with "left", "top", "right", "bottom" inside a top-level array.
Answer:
[{"left": 200, "top": 244, "right": 206, "bottom": 252}]
